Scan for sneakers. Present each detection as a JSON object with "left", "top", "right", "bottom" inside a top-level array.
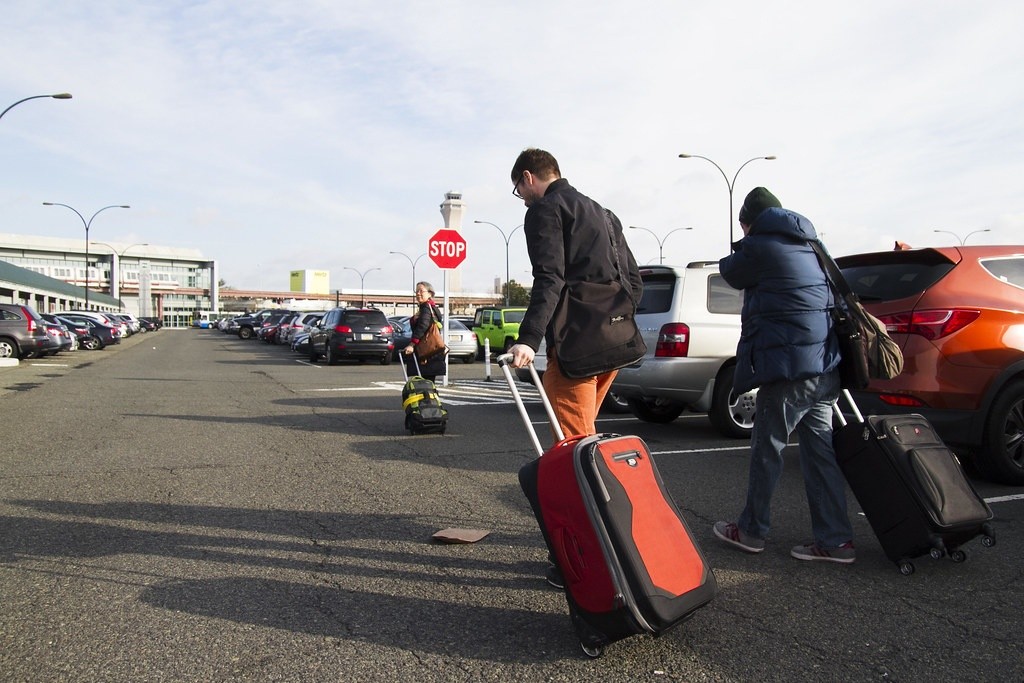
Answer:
[
  {"left": 714, "top": 520, "right": 765, "bottom": 552},
  {"left": 790, "top": 539, "right": 856, "bottom": 564}
]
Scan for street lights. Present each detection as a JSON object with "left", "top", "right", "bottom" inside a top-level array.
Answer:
[
  {"left": 629, "top": 225, "right": 693, "bottom": 265},
  {"left": 344, "top": 267, "right": 381, "bottom": 307},
  {"left": 934, "top": 229, "right": 991, "bottom": 246},
  {"left": 43, "top": 202, "right": 130, "bottom": 310},
  {"left": 91, "top": 242, "right": 149, "bottom": 313},
  {"left": 390, "top": 251, "right": 427, "bottom": 315},
  {"left": 679, "top": 153, "right": 777, "bottom": 254},
  {"left": 475, "top": 220, "right": 524, "bottom": 306}
]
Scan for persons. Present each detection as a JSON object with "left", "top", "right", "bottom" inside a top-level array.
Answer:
[
  {"left": 711, "top": 187, "right": 854, "bottom": 563},
  {"left": 507, "top": 148, "right": 643, "bottom": 588},
  {"left": 404, "top": 282, "right": 442, "bottom": 383}
]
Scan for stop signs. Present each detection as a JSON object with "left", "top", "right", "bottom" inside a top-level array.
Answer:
[{"left": 428, "top": 228, "right": 467, "bottom": 269}]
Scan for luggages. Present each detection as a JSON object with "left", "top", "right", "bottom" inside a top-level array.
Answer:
[
  {"left": 497, "top": 353, "right": 718, "bottom": 658},
  {"left": 397, "top": 349, "right": 449, "bottom": 435},
  {"left": 832, "top": 389, "right": 996, "bottom": 576}
]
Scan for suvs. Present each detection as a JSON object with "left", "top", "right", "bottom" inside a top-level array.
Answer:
[
  {"left": 308, "top": 305, "right": 396, "bottom": 366},
  {"left": 0, "top": 303, "right": 49, "bottom": 360},
  {"left": 606, "top": 259, "right": 759, "bottom": 440},
  {"left": 831, "top": 244, "right": 1024, "bottom": 488}
]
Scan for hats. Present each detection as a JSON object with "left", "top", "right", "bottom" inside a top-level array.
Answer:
[{"left": 739, "top": 187, "right": 782, "bottom": 226}]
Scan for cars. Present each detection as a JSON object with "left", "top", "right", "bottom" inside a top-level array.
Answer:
[
  {"left": 23, "top": 309, "right": 163, "bottom": 359},
  {"left": 218, "top": 307, "right": 332, "bottom": 353},
  {"left": 384, "top": 304, "right": 528, "bottom": 364}
]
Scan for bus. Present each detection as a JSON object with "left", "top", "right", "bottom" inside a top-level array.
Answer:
[{"left": 193, "top": 310, "right": 246, "bottom": 329}]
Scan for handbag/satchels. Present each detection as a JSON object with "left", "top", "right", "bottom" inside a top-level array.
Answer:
[
  {"left": 414, "top": 303, "right": 446, "bottom": 359},
  {"left": 551, "top": 280, "right": 647, "bottom": 379},
  {"left": 802, "top": 237, "right": 870, "bottom": 389},
  {"left": 807, "top": 239, "right": 905, "bottom": 380}
]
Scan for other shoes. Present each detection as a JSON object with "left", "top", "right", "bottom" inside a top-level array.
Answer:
[
  {"left": 547, "top": 551, "right": 559, "bottom": 565},
  {"left": 545, "top": 566, "right": 565, "bottom": 588}
]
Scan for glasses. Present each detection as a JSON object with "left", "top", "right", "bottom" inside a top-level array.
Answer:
[
  {"left": 414, "top": 290, "right": 428, "bottom": 294},
  {"left": 512, "top": 169, "right": 534, "bottom": 201}
]
